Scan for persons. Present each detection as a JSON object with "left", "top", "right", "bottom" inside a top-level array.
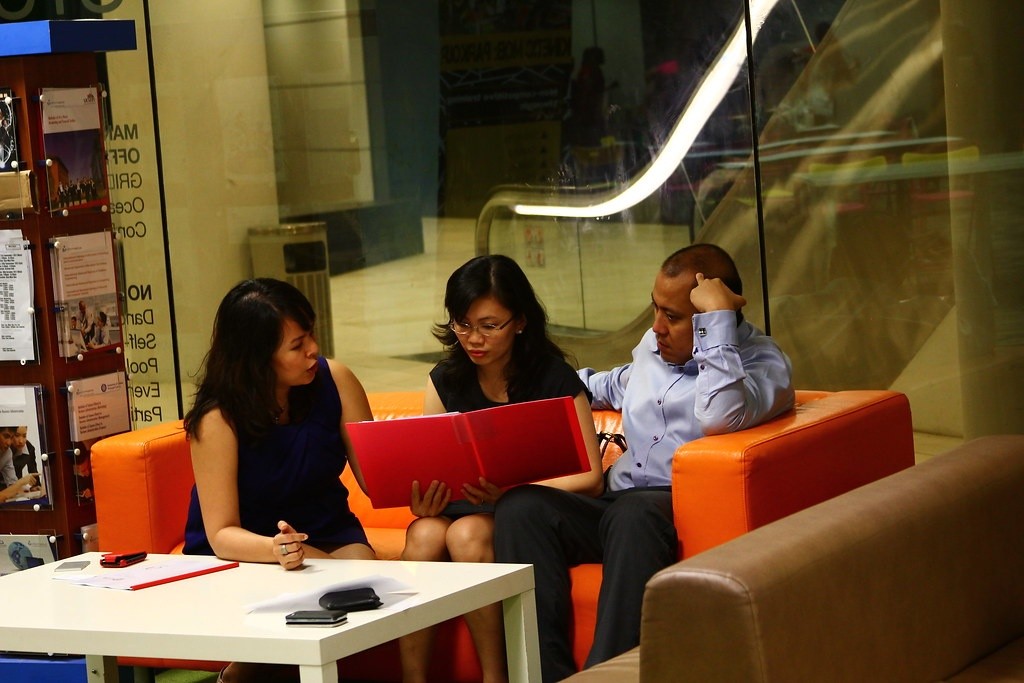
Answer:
[
  {"left": 492, "top": 243, "right": 797, "bottom": 683},
  {"left": 399, "top": 253, "right": 605, "bottom": 683},
  {"left": 0, "top": 426, "right": 42, "bottom": 504},
  {"left": 57, "top": 177, "right": 97, "bottom": 207},
  {"left": 71, "top": 300, "right": 111, "bottom": 349},
  {"left": 182, "top": 278, "right": 374, "bottom": 683},
  {"left": 645, "top": 72, "right": 672, "bottom": 146},
  {"left": 568, "top": 46, "right": 607, "bottom": 150},
  {"left": 750, "top": 18, "right": 862, "bottom": 132}
]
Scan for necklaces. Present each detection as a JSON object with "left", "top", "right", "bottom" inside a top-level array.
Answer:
[{"left": 272, "top": 403, "right": 287, "bottom": 424}]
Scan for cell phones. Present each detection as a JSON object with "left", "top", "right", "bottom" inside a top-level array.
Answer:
[{"left": 285, "top": 610, "right": 346, "bottom": 624}]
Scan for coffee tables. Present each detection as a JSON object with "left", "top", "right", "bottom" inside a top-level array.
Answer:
[{"left": 0, "top": 551, "right": 543, "bottom": 682}]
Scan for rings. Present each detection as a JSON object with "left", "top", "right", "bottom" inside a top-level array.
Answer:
[{"left": 281, "top": 545, "right": 288, "bottom": 554}]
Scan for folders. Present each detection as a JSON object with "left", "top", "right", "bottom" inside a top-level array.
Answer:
[
  {"left": 345, "top": 395, "right": 592, "bottom": 510},
  {"left": 70, "top": 558, "right": 240, "bottom": 592}
]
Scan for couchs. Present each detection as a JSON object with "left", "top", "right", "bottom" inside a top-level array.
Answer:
[
  {"left": 89, "top": 377, "right": 916, "bottom": 683},
  {"left": 554, "top": 427, "right": 1024, "bottom": 683}
]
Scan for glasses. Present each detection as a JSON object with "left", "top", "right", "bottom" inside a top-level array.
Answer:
[{"left": 448, "top": 315, "right": 515, "bottom": 336}]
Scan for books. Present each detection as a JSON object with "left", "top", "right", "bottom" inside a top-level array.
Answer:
[
  {"left": 48, "top": 231, "right": 121, "bottom": 357},
  {"left": 0, "top": 385, "right": 54, "bottom": 512},
  {"left": 66, "top": 372, "right": 130, "bottom": 503},
  {"left": 81, "top": 523, "right": 99, "bottom": 554},
  {"left": 0, "top": 535, "right": 56, "bottom": 577},
  {"left": 38, "top": 87, "right": 110, "bottom": 217}
]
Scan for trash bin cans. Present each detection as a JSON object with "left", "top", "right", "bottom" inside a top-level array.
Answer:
[
  {"left": 444, "top": 118, "right": 567, "bottom": 219},
  {"left": 247, "top": 221, "right": 338, "bottom": 361}
]
